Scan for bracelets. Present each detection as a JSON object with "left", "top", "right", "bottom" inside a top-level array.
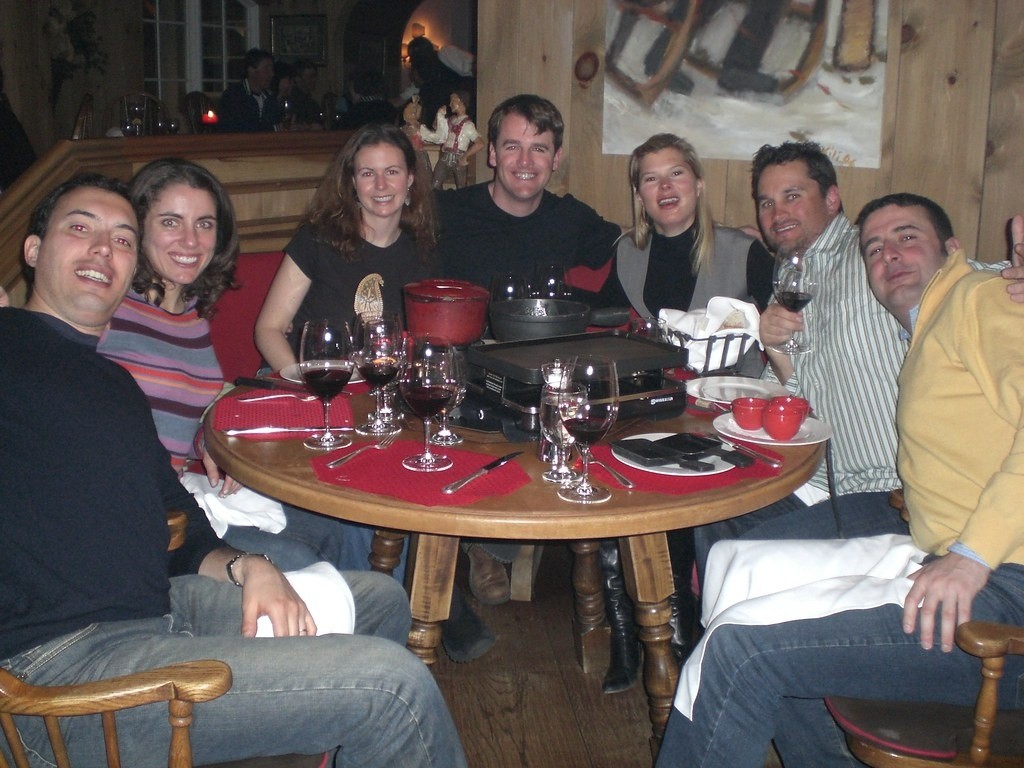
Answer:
[{"left": 225, "top": 551, "right": 273, "bottom": 588}]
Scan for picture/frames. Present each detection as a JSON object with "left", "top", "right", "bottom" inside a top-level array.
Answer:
[
  {"left": 362, "top": 30, "right": 388, "bottom": 80},
  {"left": 270, "top": 15, "right": 329, "bottom": 68}
]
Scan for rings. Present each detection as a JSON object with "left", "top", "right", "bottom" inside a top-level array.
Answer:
[{"left": 299, "top": 628, "right": 307, "bottom": 631}]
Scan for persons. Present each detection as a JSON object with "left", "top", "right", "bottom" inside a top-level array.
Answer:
[
  {"left": 1, "top": 173, "right": 469, "bottom": 767},
  {"left": 214, "top": 37, "right": 479, "bottom": 130},
  {"left": 648, "top": 189, "right": 1024, "bottom": 768},
  {"left": 399, "top": 89, "right": 485, "bottom": 189},
  {"left": 592, "top": 133, "right": 778, "bottom": 696},
  {"left": 406, "top": 94, "right": 776, "bottom": 608},
  {"left": 253, "top": 122, "right": 499, "bottom": 661},
  {"left": 691, "top": 142, "right": 1024, "bottom": 596},
  {"left": 90, "top": 156, "right": 417, "bottom": 649}
]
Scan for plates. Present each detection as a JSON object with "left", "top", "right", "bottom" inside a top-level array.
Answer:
[
  {"left": 713, "top": 411, "right": 833, "bottom": 445},
  {"left": 279, "top": 360, "right": 368, "bottom": 386},
  {"left": 610, "top": 432, "right": 739, "bottom": 476},
  {"left": 683, "top": 376, "right": 791, "bottom": 406}
]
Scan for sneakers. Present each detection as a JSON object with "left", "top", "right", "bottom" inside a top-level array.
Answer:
[{"left": 441, "top": 584, "right": 495, "bottom": 662}]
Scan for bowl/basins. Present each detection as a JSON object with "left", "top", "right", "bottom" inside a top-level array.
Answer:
[
  {"left": 403, "top": 278, "right": 489, "bottom": 345},
  {"left": 488, "top": 298, "right": 590, "bottom": 341}
]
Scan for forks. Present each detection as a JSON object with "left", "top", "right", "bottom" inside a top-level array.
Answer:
[
  {"left": 326, "top": 426, "right": 404, "bottom": 469},
  {"left": 237, "top": 393, "right": 319, "bottom": 404},
  {"left": 574, "top": 440, "right": 635, "bottom": 488}
]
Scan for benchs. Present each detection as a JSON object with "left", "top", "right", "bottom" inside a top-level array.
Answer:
[{"left": 206, "top": 251, "right": 646, "bottom": 384}]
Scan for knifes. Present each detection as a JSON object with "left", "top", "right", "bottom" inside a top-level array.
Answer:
[
  {"left": 221, "top": 423, "right": 353, "bottom": 435},
  {"left": 705, "top": 430, "right": 784, "bottom": 468},
  {"left": 440, "top": 451, "right": 526, "bottom": 493},
  {"left": 235, "top": 375, "right": 313, "bottom": 394}
]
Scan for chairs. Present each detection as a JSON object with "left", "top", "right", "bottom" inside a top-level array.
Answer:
[
  {"left": 72, "top": 91, "right": 216, "bottom": 139},
  {"left": 824, "top": 488, "right": 1024, "bottom": 767},
  {"left": 1, "top": 511, "right": 233, "bottom": 768}
]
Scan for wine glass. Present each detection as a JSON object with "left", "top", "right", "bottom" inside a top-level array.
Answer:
[
  {"left": 353, "top": 312, "right": 463, "bottom": 473},
  {"left": 300, "top": 320, "right": 354, "bottom": 451},
  {"left": 538, "top": 353, "right": 614, "bottom": 505},
  {"left": 766, "top": 246, "right": 818, "bottom": 356}
]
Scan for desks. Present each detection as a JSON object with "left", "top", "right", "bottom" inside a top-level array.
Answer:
[{"left": 205, "top": 353, "right": 825, "bottom": 768}]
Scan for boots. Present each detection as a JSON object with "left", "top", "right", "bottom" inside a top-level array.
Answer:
[
  {"left": 667, "top": 537, "right": 694, "bottom": 662},
  {"left": 598, "top": 543, "right": 642, "bottom": 694}
]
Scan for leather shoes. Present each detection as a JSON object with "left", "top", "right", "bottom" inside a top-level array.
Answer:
[{"left": 460, "top": 537, "right": 511, "bottom": 605}]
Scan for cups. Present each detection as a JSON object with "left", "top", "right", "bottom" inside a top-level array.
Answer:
[
  {"left": 120, "top": 100, "right": 179, "bottom": 138},
  {"left": 490, "top": 265, "right": 576, "bottom": 307},
  {"left": 631, "top": 317, "right": 668, "bottom": 344}
]
